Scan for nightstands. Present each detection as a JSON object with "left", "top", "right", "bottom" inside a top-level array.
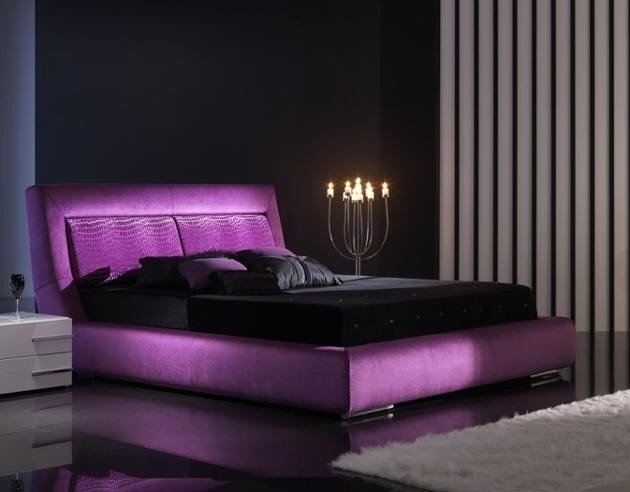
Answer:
[{"left": 1, "top": 311, "right": 73, "bottom": 396}]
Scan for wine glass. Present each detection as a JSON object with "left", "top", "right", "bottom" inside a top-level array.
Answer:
[{"left": 8, "top": 273, "right": 25, "bottom": 320}]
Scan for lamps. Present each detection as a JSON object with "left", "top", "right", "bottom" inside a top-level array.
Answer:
[{"left": 326, "top": 178, "right": 392, "bottom": 275}]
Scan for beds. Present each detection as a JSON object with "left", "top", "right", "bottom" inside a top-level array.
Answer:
[{"left": 24, "top": 182, "right": 576, "bottom": 419}]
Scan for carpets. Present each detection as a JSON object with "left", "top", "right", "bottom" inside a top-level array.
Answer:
[{"left": 328, "top": 389, "right": 630, "bottom": 492}]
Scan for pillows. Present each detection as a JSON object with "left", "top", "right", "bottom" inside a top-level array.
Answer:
[{"left": 178, "top": 246, "right": 342, "bottom": 293}]
